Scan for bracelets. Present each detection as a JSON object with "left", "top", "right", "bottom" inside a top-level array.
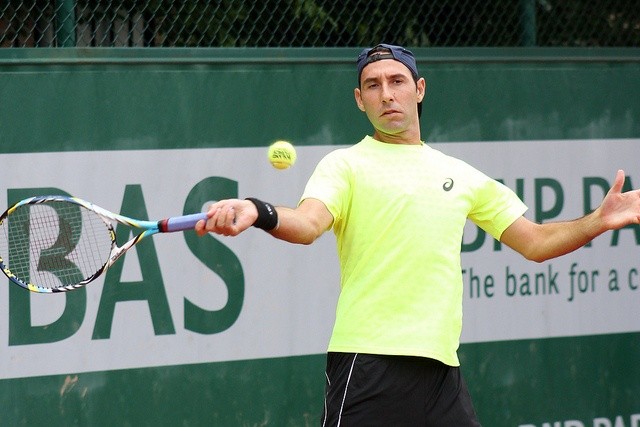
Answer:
[{"left": 245, "top": 195, "right": 280, "bottom": 233}]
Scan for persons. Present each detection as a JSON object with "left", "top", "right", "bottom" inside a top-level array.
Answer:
[{"left": 194, "top": 44, "right": 640, "bottom": 425}]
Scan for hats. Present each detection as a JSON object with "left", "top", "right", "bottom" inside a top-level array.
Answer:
[{"left": 356, "top": 43, "right": 422, "bottom": 118}]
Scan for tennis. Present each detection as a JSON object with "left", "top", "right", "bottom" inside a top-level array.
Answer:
[{"left": 269, "top": 141, "right": 296, "bottom": 169}]
